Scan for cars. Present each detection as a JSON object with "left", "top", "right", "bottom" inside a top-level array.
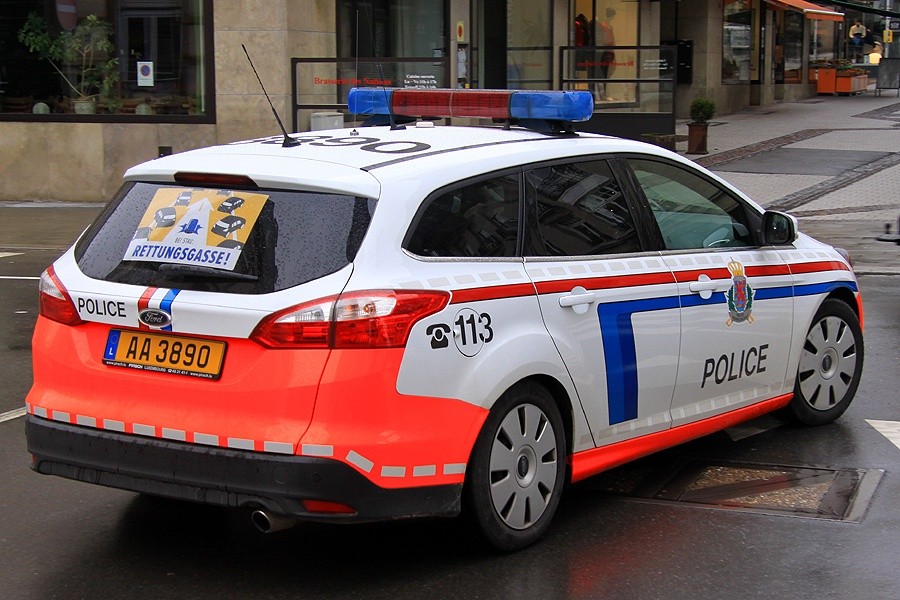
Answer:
[{"left": 23, "top": 81, "right": 867, "bottom": 552}]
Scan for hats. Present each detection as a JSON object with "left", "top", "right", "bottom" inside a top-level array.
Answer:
[{"left": 606, "top": 6, "right": 616, "bottom": 17}]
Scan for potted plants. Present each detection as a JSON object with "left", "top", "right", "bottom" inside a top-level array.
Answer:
[
  {"left": 17, "top": 10, "right": 121, "bottom": 115},
  {"left": 686, "top": 99, "right": 716, "bottom": 154}
]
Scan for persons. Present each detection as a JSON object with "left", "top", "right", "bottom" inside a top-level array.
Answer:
[
  {"left": 574, "top": 6, "right": 617, "bottom": 100},
  {"left": 849, "top": 17, "right": 866, "bottom": 58}
]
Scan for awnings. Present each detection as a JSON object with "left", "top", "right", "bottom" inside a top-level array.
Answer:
[
  {"left": 765, "top": 0, "right": 845, "bottom": 22},
  {"left": 807, "top": 0, "right": 900, "bottom": 18}
]
